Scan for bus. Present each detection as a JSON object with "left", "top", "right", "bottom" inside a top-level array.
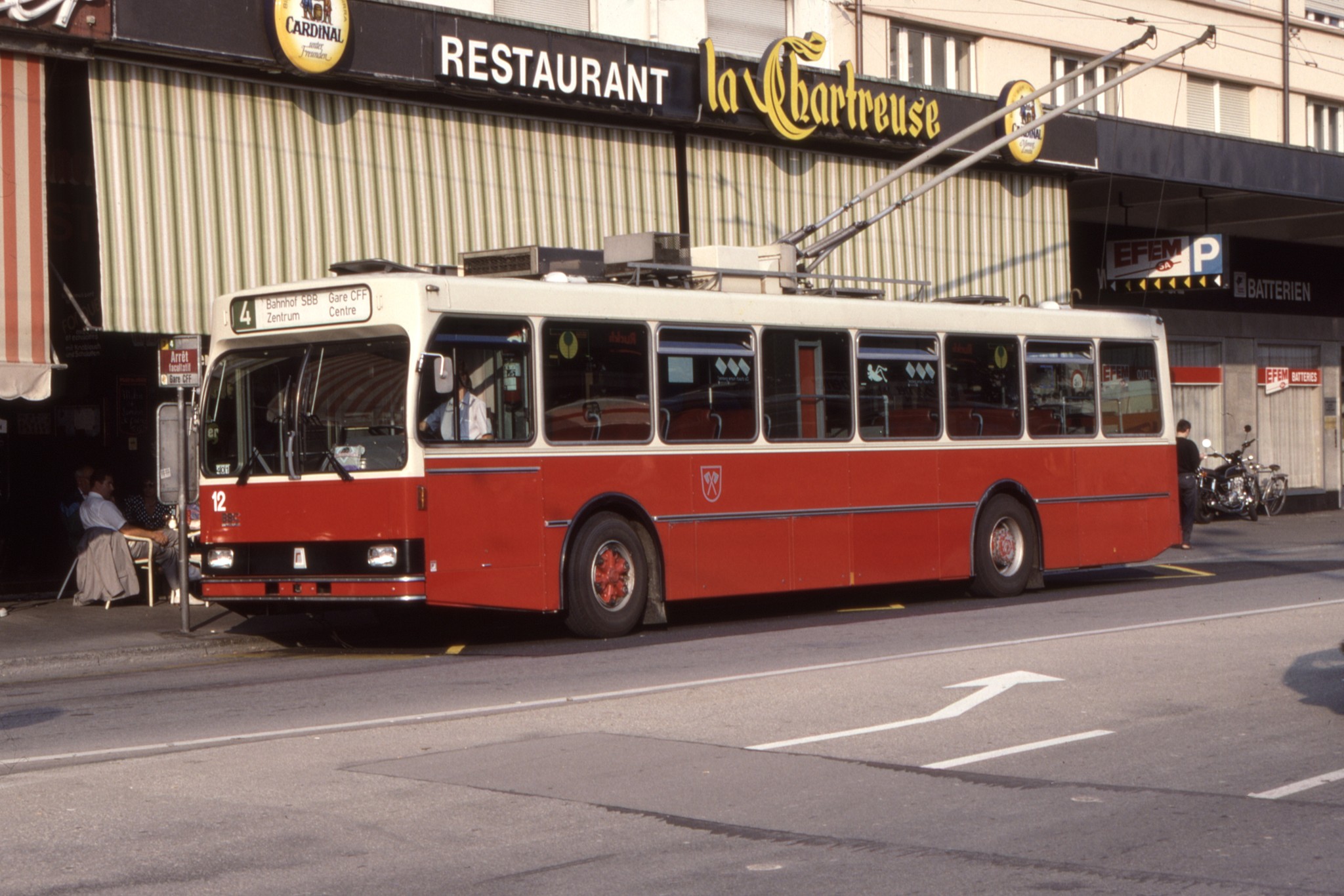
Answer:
[{"left": 192, "top": 22, "right": 1184, "bottom": 640}]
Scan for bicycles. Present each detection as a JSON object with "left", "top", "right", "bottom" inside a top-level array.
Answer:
[{"left": 1254, "top": 464, "right": 1289, "bottom": 517}]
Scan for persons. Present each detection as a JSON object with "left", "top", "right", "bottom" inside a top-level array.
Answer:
[
  {"left": 418, "top": 354, "right": 494, "bottom": 440},
  {"left": 122, "top": 471, "right": 173, "bottom": 530},
  {"left": 1176, "top": 418, "right": 1200, "bottom": 549},
  {"left": 79, "top": 468, "right": 205, "bottom": 606},
  {"left": 67, "top": 466, "right": 96, "bottom": 542}
]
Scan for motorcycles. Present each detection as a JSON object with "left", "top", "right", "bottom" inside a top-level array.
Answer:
[{"left": 1192, "top": 424, "right": 1260, "bottom": 524}]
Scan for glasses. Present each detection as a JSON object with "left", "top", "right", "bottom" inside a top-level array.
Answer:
[{"left": 142, "top": 484, "right": 154, "bottom": 488}]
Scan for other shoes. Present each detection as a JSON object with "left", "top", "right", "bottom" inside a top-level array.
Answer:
[
  {"left": 171, "top": 588, "right": 205, "bottom": 605},
  {"left": 187, "top": 564, "right": 202, "bottom": 582},
  {"left": 1182, "top": 542, "right": 1190, "bottom": 550}
]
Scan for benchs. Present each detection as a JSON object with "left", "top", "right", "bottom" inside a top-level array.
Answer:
[{"left": 548, "top": 395, "right": 1158, "bottom": 441}]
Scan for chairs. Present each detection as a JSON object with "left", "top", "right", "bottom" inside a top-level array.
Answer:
[{"left": 56, "top": 502, "right": 175, "bottom": 610}]
